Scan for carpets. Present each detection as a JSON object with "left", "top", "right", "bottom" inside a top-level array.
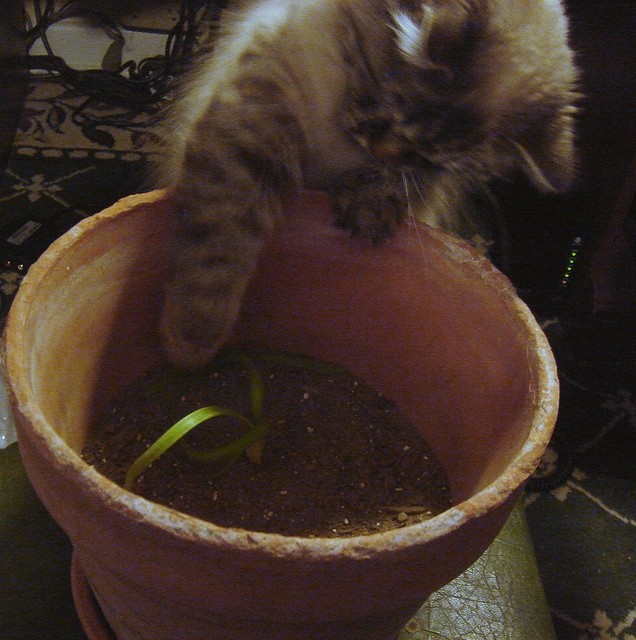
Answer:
[{"left": 0, "top": 72, "right": 636, "bottom": 640}]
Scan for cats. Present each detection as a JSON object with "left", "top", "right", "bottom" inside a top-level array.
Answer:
[{"left": 155, "top": 0, "right": 581, "bottom": 369}]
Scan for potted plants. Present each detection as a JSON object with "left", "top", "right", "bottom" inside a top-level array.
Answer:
[{"left": 0, "top": 185, "right": 562, "bottom": 640}]
